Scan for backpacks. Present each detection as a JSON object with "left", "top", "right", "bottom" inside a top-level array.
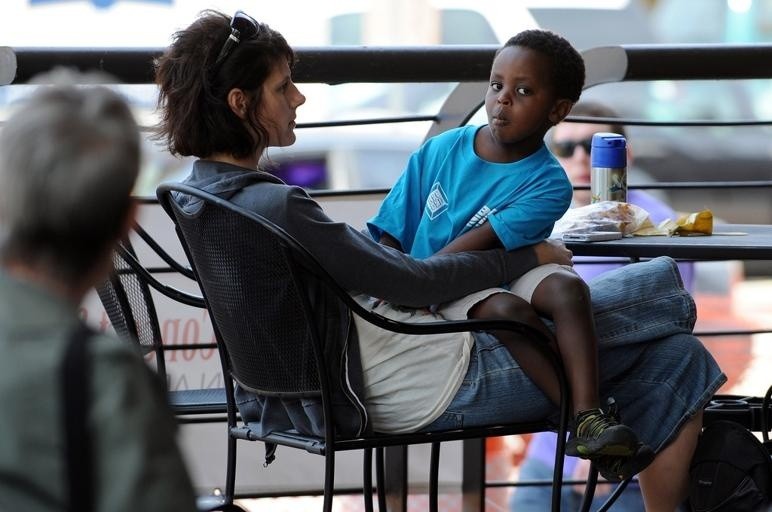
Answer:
[{"left": 681, "top": 419, "right": 772, "bottom": 512}]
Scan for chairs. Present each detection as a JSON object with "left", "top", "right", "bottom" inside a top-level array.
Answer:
[
  {"left": 90, "top": 198, "right": 241, "bottom": 512},
  {"left": 158, "top": 180, "right": 571, "bottom": 512}
]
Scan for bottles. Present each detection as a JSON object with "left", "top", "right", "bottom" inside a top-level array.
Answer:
[{"left": 589, "top": 131, "right": 628, "bottom": 204}]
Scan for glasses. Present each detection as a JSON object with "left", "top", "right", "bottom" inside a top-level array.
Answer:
[
  {"left": 208, "top": 11, "right": 261, "bottom": 89},
  {"left": 551, "top": 140, "right": 591, "bottom": 158}
]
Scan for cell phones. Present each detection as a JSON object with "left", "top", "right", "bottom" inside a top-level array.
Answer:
[{"left": 562, "top": 231, "right": 622, "bottom": 243}]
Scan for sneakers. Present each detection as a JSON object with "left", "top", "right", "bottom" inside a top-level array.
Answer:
[
  {"left": 596, "top": 442, "right": 656, "bottom": 483},
  {"left": 565, "top": 396, "right": 638, "bottom": 459}
]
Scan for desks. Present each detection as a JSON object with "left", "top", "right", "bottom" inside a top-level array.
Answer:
[{"left": 550, "top": 223, "right": 771, "bottom": 512}]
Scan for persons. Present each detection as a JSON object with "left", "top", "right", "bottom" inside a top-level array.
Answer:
[
  {"left": 363, "top": 28, "right": 656, "bottom": 486},
  {"left": 156, "top": 13, "right": 724, "bottom": 512},
  {"left": 493, "top": 99, "right": 695, "bottom": 512},
  {"left": 1, "top": 79, "right": 195, "bottom": 511}
]
